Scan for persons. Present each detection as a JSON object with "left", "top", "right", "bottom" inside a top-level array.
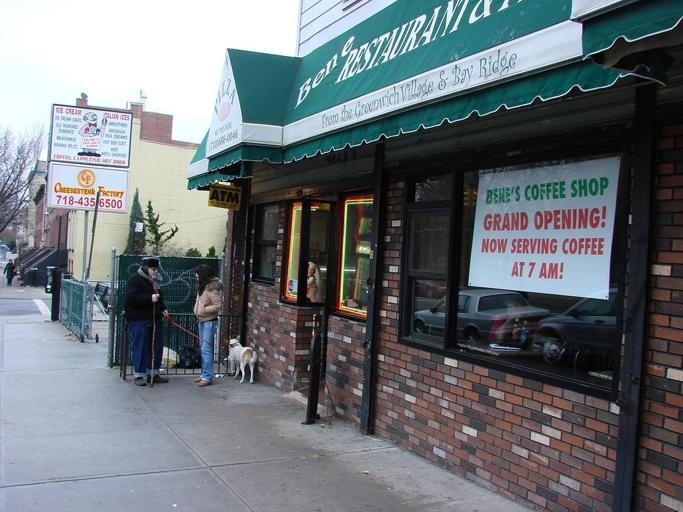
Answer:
[
  {"left": 488, "top": 297, "right": 529, "bottom": 342},
  {"left": 192, "top": 263, "right": 223, "bottom": 386},
  {"left": 123, "top": 257, "right": 169, "bottom": 386},
  {"left": 3, "top": 259, "right": 14, "bottom": 286}
]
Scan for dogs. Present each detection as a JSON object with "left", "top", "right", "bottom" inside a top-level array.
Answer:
[{"left": 224, "top": 334, "right": 258, "bottom": 384}]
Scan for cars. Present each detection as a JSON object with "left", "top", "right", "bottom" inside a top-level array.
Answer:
[
  {"left": 414, "top": 288, "right": 549, "bottom": 348},
  {"left": 95, "top": 270, "right": 196, "bottom": 312},
  {"left": 533, "top": 287, "right": 618, "bottom": 366}
]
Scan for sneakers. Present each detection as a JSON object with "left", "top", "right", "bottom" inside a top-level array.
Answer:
[
  {"left": 194, "top": 377, "right": 210, "bottom": 386},
  {"left": 146, "top": 374, "right": 169, "bottom": 383},
  {"left": 133, "top": 376, "right": 145, "bottom": 385}
]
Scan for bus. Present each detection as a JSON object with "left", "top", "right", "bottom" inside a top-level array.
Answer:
[{"left": 0, "top": 245, "right": 10, "bottom": 271}]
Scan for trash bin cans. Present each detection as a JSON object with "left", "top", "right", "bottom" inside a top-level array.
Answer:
[
  {"left": 30, "top": 268, "right": 37, "bottom": 286},
  {"left": 44, "top": 266, "right": 69, "bottom": 292}
]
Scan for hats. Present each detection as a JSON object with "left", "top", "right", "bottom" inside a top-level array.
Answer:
[{"left": 140, "top": 257, "right": 159, "bottom": 268}]
